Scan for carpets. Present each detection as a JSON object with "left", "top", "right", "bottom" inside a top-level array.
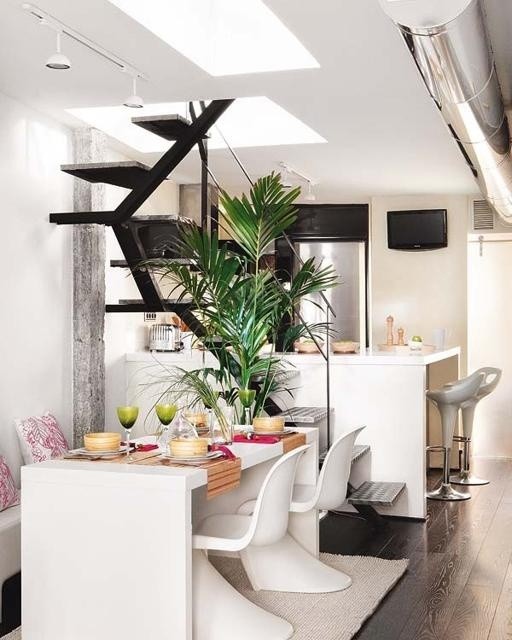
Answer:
[{"left": 0, "top": 550, "right": 412, "bottom": 640}]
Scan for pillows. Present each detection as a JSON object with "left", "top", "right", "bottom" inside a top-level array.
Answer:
[
  {"left": 13, "top": 409, "right": 73, "bottom": 463},
  {"left": 0, "top": 458, "right": 18, "bottom": 515}
]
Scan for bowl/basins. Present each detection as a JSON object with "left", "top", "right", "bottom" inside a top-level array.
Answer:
[
  {"left": 331, "top": 342, "right": 360, "bottom": 355},
  {"left": 183, "top": 413, "right": 208, "bottom": 426},
  {"left": 167, "top": 437, "right": 208, "bottom": 457},
  {"left": 84, "top": 431, "right": 122, "bottom": 450},
  {"left": 407, "top": 340, "right": 422, "bottom": 349},
  {"left": 252, "top": 417, "right": 285, "bottom": 433},
  {"left": 293, "top": 341, "right": 325, "bottom": 355}
]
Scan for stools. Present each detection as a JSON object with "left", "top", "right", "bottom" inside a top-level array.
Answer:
[
  {"left": 442, "top": 365, "right": 502, "bottom": 488},
  {"left": 422, "top": 367, "right": 487, "bottom": 502}
]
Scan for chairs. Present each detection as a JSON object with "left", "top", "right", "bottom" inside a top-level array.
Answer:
[
  {"left": 189, "top": 443, "right": 315, "bottom": 640},
  {"left": 234, "top": 423, "right": 370, "bottom": 596}
]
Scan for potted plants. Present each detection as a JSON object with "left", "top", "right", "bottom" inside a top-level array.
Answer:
[{"left": 122, "top": 171, "right": 344, "bottom": 425}]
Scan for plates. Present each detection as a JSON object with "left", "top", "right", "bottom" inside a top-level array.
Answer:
[
  {"left": 156, "top": 450, "right": 225, "bottom": 466},
  {"left": 379, "top": 343, "right": 435, "bottom": 352},
  {"left": 76, "top": 448, "right": 126, "bottom": 453},
  {"left": 70, "top": 447, "right": 137, "bottom": 461},
  {"left": 243, "top": 427, "right": 300, "bottom": 437},
  {"left": 196, "top": 426, "right": 208, "bottom": 429},
  {"left": 196, "top": 429, "right": 211, "bottom": 435},
  {"left": 248, "top": 429, "right": 291, "bottom": 433}
]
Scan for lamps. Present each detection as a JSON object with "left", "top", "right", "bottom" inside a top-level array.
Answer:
[
  {"left": 122, "top": 91, "right": 147, "bottom": 108},
  {"left": 46, "top": 54, "right": 72, "bottom": 71}
]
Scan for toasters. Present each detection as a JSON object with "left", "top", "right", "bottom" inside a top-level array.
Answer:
[{"left": 147, "top": 323, "right": 183, "bottom": 352}]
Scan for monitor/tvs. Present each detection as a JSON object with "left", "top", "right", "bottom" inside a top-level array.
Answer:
[{"left": 386, "top": 210, "right": 447, "bottom": 252}]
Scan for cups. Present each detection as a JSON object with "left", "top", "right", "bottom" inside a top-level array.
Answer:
[
  {"left": 432, "top": 327, "right": 444, "bottom": 350},
  {"left": 163, "top": 451, "right": 220, "bottom": 459},
  {"left": 155, "top": 403, "right": 177, "bottom": 455},
  {"left": 210, "top": 406, "right": 234, "bottom": 445},
  {"left": 117, "top": 403, "right": 139, "bottom": 461},
  {"left": 237, "top": 389, "right": 257, "bottom": 438}
]
